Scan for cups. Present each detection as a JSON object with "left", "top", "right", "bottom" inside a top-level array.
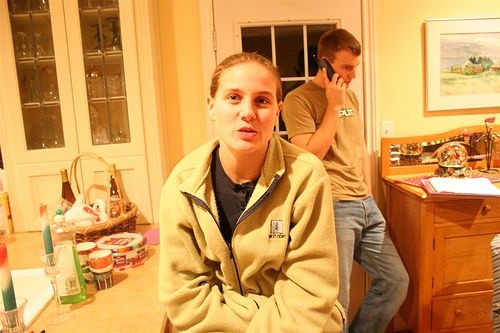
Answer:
[
  {"left": 76, "top": 242, "right": 97, "bottom": 288},
  {"left": 88, "top": 250, "right": 113, "bottom": 290}
]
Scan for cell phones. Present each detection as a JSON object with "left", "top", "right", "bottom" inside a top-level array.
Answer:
[{"left": 312, "top": 54, "right": 336, "bottom": 81}]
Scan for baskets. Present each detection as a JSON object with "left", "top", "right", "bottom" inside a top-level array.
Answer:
[{"left": 69, "top": 154, "right": 138, "bottom": 244}]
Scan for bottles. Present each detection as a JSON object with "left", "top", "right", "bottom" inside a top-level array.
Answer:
[
  {"left": 108, "top": 164, "right": 122, "bottom": 218},
  {"left": 60, "top": 169, "right": 76, "bottom": 214},
  {"left": 52, "top": 210, "right": 88, "bottom": 304}
]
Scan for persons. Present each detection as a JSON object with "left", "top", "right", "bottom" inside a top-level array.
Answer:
[
  {"left": 283, "top": 28, "right": 409, "bottom": 333},
  {"left": 159, "top": 52, "right": 346, "bottom": 333}
]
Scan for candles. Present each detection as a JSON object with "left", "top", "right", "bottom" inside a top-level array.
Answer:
[
  {"left": 0, "top": 241, "right": 17, "bottom": 310},
  {"left": 38, "top": 200, "right": 55, "bottom": 266}
]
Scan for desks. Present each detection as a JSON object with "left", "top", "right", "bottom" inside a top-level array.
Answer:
[{"left": 0, "top": 224, "right": 176, "bottom": 333}]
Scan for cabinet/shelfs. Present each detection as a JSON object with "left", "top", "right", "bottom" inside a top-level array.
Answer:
[{"left": 380, "top": 125, "right": 500, "bottom": 332}]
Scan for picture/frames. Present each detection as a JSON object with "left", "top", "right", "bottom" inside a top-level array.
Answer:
[{"left": 420, "top": 17, "right": 500, "bottom": 117}]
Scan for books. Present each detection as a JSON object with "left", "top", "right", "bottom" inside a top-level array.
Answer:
[{"left": 420, "top": 177, "right": 500, "bottom": 196}]
